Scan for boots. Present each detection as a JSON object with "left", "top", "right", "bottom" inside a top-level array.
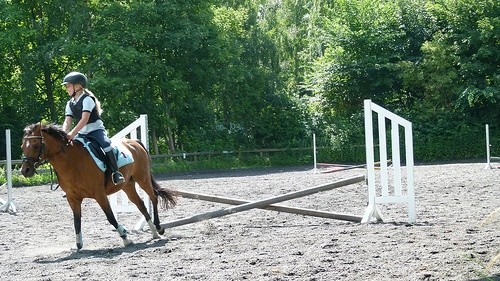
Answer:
[{"left": 105, "top": 150, "right": 124, "bottom": 183}]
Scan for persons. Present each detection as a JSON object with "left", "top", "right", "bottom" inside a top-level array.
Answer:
[{"left": 61, "top": 72, "right": 125, "bottom": 185}]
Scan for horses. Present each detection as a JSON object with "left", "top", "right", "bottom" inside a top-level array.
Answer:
[{"left": 21, "top": 122, "right": 177, "bottom": 252}]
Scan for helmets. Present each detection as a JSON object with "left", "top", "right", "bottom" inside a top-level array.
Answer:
[{"left": 61, "top": 72, "right": 87, "bottom": 88}]
